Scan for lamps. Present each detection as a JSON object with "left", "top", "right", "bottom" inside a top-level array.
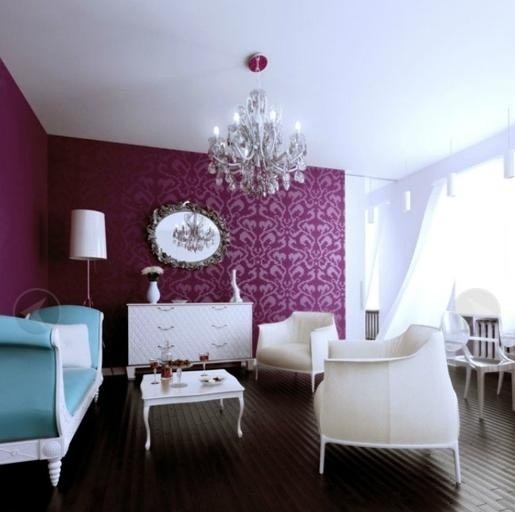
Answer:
[
  {"left": 208, "top": 52, "right": 308, "bottom": 200},
  {"left": 172, "top": 211, "right": 219, "bottom": 252},
  {"left": 68, "top": 209, "right": 108, "bottom": 308}
]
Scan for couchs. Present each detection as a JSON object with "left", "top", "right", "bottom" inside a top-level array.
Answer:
[{"left": 0, "top": 304, "right": 104, "bottom": 487}]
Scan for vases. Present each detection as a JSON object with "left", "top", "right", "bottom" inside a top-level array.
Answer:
[{"left": 146, "top": 281, "right": 161, "bottom": 304}]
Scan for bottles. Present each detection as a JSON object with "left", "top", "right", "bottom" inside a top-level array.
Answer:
[{"left": 161, "top": 340, "right": 173, "bottom": 380}]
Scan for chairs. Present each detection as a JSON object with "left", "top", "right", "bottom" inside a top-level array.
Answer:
[
  {"left": 463, "top": 318, "right": 515, "bottom": 420},
  {"left": 313, "top": 324, "right": 461, "bottom": 486},
  {"left": 254, "top": 311, "right": 340, "bottom": 393}
]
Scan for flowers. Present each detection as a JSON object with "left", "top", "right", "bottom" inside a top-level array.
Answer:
[{"left": 140, "top": 265, "right": 164, "bottom": 282}]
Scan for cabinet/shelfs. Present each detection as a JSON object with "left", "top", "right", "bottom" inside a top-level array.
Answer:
[{"left": 126, "top": 301, "right": 254, "bottom": 380}]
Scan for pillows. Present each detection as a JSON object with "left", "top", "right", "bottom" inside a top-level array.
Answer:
[{"left": 56, "top": 324, "right": 92, "bottom": 369}]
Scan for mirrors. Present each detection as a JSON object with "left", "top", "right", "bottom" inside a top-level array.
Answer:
[{"left": 144, "top": 200, "right": 230, "bottom": 271}]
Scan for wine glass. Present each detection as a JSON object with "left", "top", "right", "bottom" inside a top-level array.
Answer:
[
  {"left": 149, "top": 358, "right": 160, "bottom": 384},
  {"left": 199, "top": 352, "right": 208, "bottom": 376}
]
